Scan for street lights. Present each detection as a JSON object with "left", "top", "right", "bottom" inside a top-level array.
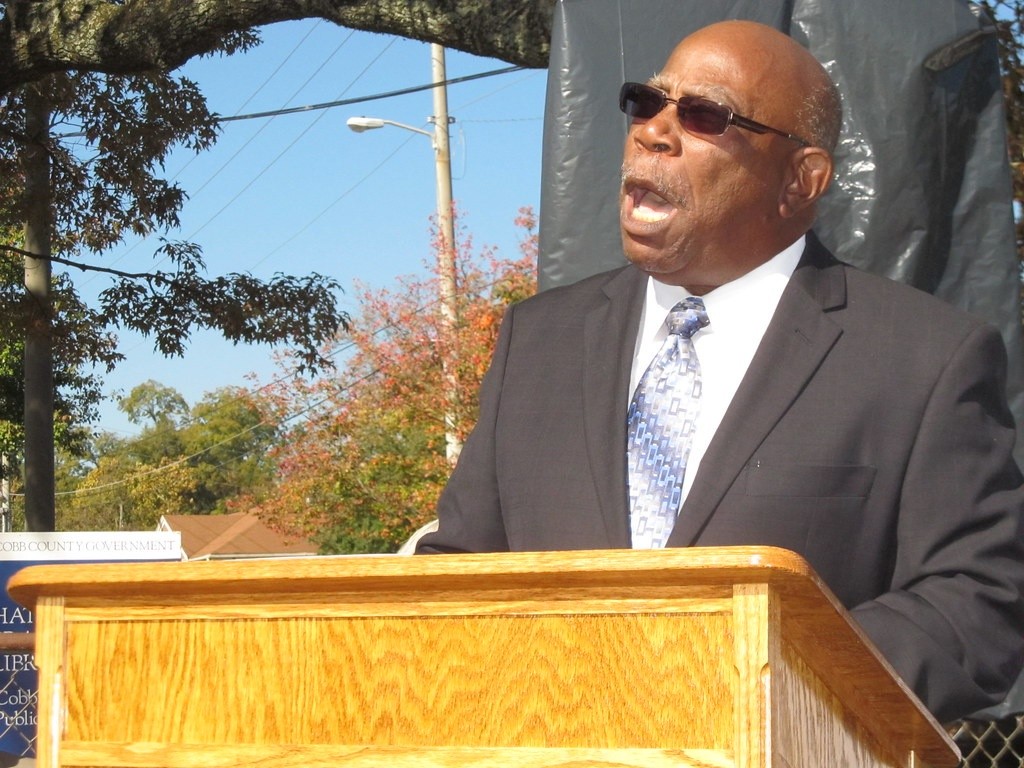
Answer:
[{"left": 341, "top": 25, "right": 462, "bottom": 468}]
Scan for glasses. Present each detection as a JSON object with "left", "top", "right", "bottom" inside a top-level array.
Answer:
[{"left": 619, "top": 82, "right": 811, "bottom": 147}]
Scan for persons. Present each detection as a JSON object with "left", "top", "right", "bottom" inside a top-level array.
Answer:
[{"left": 412, "top": 17, "right": 1024, "bottom": 723}]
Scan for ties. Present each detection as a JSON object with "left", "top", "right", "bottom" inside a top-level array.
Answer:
[{"left": 627, "top": 297, "right": 709, "bottom": 549}]
